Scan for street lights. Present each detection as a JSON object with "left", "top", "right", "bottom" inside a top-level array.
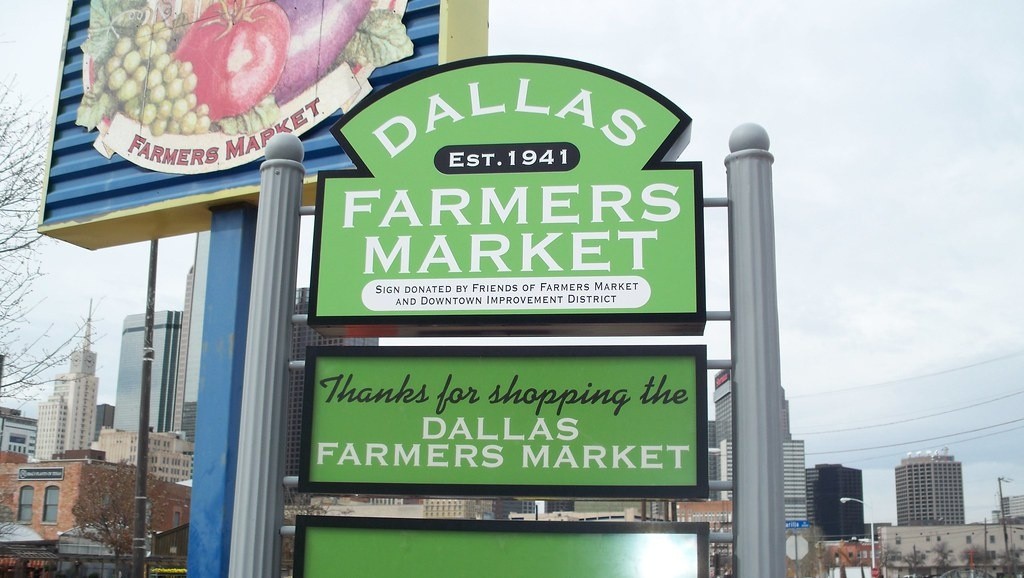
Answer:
[{"left": 840, "top": 498, "right": 876, "bottom": 570}]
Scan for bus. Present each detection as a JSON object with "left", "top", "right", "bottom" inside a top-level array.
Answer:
[{"left": 149, "top": 567, "right": 187, "bottom": 578}]
